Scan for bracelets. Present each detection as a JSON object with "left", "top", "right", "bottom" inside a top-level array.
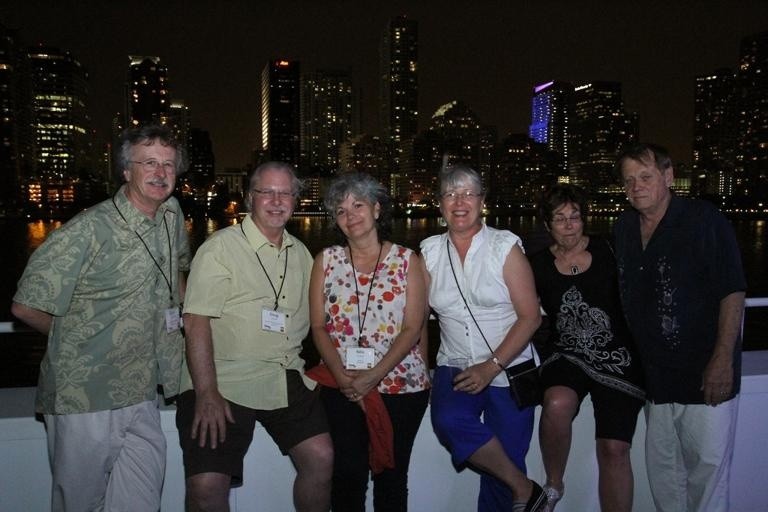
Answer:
[{"left": 489, "top": 355, "right": 506, "bottom": 371}]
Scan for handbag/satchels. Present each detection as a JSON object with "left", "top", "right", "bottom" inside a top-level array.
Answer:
[{"left": 506, "top": 358, "right": 543, "bottom": 412}]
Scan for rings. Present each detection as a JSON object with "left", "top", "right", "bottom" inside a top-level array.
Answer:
[
  {"left": 352, "top": 392, "right": 357, "bottom": 399},
  {"left": 720, "top": 391, "right": 728, "bottom": 395}
]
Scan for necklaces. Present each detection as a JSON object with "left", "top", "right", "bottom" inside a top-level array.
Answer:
[{"left": 556, "top": 235, "right": 592, "bottom": 276}]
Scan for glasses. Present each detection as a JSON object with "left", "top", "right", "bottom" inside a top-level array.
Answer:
[
  {"left": 440, "top": 190, "right": 482, "bottom": 202},
  {"left": 252, "top": 188, "right": 294, "bottom": 202}
]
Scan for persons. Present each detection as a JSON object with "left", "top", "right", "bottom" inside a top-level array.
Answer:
[
  {"left": 414, "top": 160, "right": 550, "bottom": 511},
  {"left": 526, "top": 182, "right": 648, "bottom": 512},
  {"left": 306, "top": 169, "right": 433, "bottom": 512},
  {"left": 610, "top": 138, "right": 749, "bottom": 511},
  {"left": 10, "top": 123, "right": 193, "bottom": 512},
  {"left": 172, "top": 157, "right": 338, "bottom": 512}
]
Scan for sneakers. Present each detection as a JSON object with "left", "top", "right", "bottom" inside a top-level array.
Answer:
[
  {"left": 538, "top": 481, "right": 564, "bottom": 512},
  {"left": 512, "top": 480, "right": 548, "bottom": 512}
]
